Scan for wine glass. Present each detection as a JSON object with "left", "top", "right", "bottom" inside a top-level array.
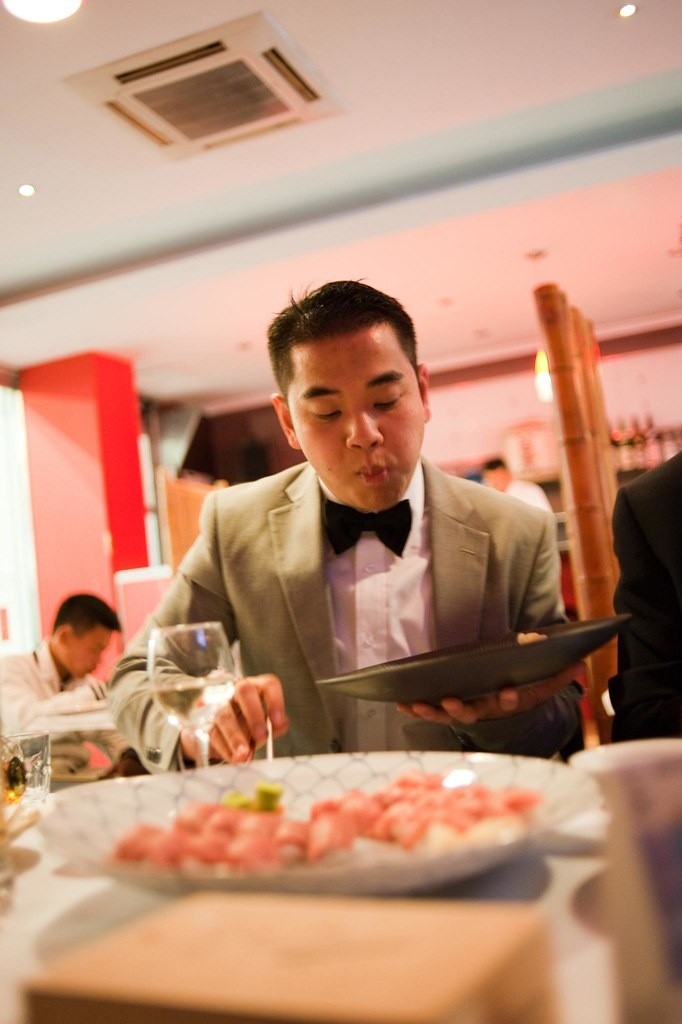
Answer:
[{"left": 148, "top": 620, "right": 237, "bottom": 768}]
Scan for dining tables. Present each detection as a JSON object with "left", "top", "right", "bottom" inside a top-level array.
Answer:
[{"left": 0, "top": 701, "right": 682, "bottom": 1024}]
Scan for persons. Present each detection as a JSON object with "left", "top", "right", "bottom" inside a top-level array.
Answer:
[
  {"left": 483, "top": 459, "right": 553, "bottom": 511},
  {"left": 607, "top": 449, "right": 682, "bottom": 740},
  {"left": 0, "top": 593, "right": 121, "bottom": 739},
  {"left": 108, "top": 282, "right": 584, "bottom": 758}
]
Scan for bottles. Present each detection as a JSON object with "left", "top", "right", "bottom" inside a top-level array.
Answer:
[
  {"left": 0, "top": 719, "right": 26, "bottom": 807},
  {"left": 606, "top": 423, "right": 618, "bottom": 471},
  {"left": 630, "top": 414, "right": 647, "bottom": 469},
  {"left": 642, "top": 413, "right": 661, "bottom": 468},
  {"left": 616, "top": 419, "right": 634, "bottom": 472}
]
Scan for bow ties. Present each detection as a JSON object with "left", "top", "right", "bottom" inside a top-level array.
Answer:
[{"left": 322, "top": 499, "right": 413, "bottom": 556}]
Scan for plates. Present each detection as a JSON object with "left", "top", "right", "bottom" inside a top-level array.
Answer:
[
  {"left": 35, "top": 751, "right": 604, "bottom": 897},
  {"left": 316, "top": 613, "right": 632, "bottom": 706}
]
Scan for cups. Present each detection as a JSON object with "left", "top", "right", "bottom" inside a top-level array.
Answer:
[
  {"left": 571, "top": 739, "right": 682, "bottom": 986},
  {"left": 4, "top": 731, "right": 52, "bottom": 807}
]
